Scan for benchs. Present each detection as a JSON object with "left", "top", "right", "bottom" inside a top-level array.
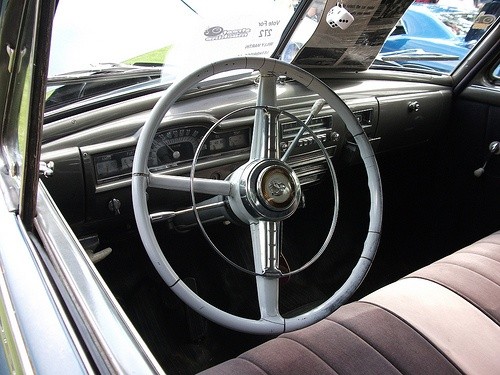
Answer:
[{"left": 161, "top": 224, "right": 499, "bottom": 375}]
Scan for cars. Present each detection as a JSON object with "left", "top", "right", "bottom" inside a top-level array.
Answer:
[{"left": 283, "top": 0, "right": 500, "bottom": 91}]
